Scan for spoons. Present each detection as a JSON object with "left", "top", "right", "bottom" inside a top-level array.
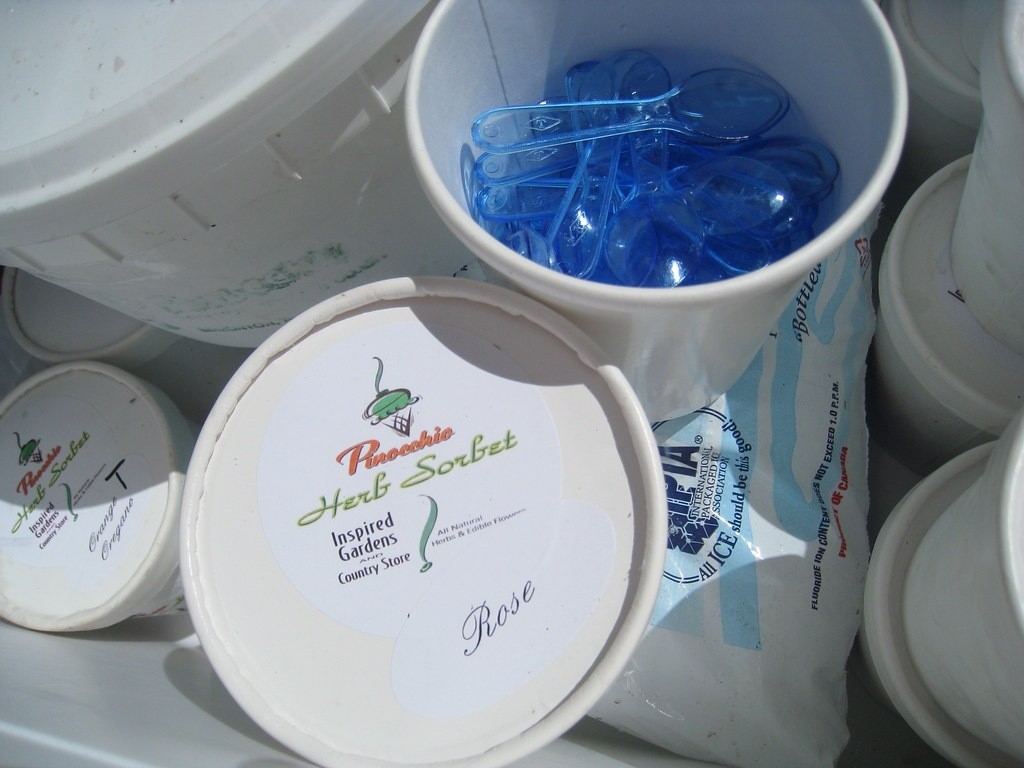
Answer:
[{"left": 471, "top": 48, "right": 841, "bottom": 287}]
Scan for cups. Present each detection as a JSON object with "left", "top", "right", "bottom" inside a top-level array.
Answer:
[{"left": 0, "top": 0, "right": 1024, "bottom": 768}]
